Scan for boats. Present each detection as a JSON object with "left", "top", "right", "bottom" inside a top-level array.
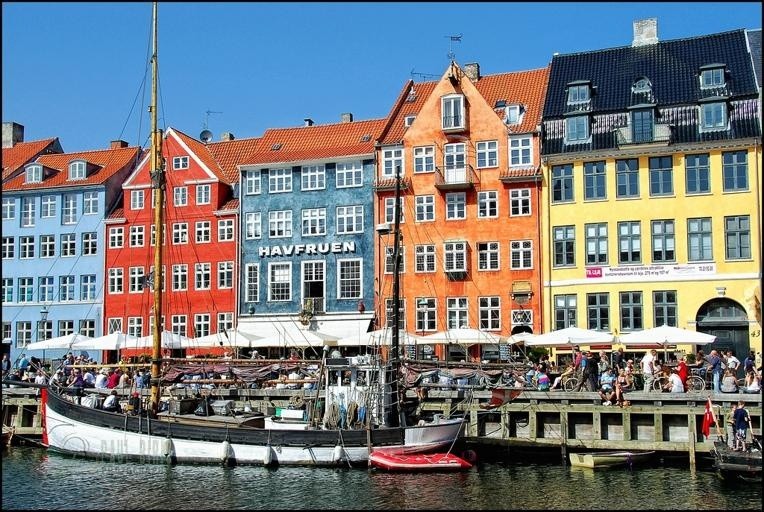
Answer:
[
  {"left": 708, "top": 442, "right": 763, "bottom": 487},
  {"left": 569, "top": 445, "right": 655, "bottom": 471}
]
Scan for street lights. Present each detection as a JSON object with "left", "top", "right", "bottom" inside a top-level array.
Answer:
[{"left": 38, "top": 305, "right": 49, "bottom": 366}]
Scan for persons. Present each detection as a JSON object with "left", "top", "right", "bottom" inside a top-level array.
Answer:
[
  {"left": 731, "top": 400, "right": 751, "bottom": 452},
  {"left": 725, "top": 404, "right": 737, "bottom": 450},
  {"left": 1, "top": 348, "right": 762, "bottom": 421}
]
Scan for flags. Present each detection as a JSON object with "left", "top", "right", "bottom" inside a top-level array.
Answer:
[{"left": 701, "top": 402, "right": 714, "bottom": 440}]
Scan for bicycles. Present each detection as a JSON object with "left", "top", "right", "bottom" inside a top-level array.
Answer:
[
  {"left": 652, "top": 371, "right": 705, "bottom": 395},
  {"left": 564, "top": 375, "right": 587, "bottom": 393}
]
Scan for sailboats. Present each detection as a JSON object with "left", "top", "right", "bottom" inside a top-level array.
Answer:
[{"left": 38, "top": 3, "right": 483, "bottom": 468}]
